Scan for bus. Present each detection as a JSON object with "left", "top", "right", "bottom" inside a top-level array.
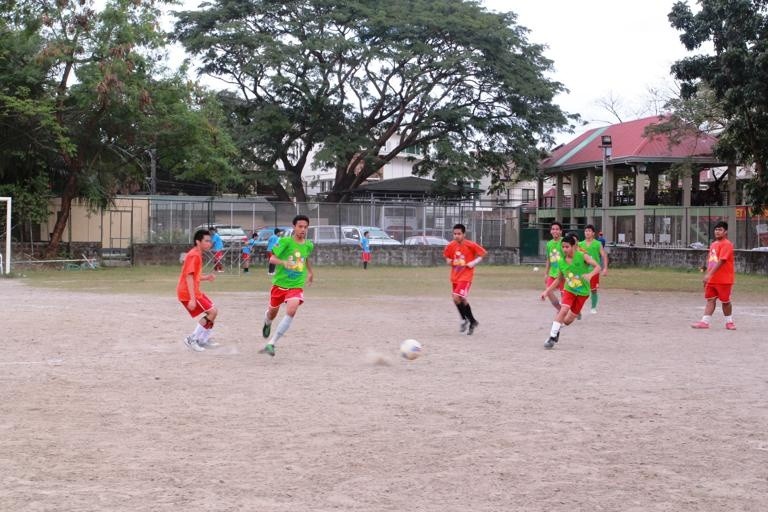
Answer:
[{"left": 379, "top": 205, "right": 418, "bottom": 236}]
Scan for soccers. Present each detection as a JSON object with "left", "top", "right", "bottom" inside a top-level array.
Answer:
[
  {"left": 533, "top": 267, "right": 540, "bottom": 271},
  {"left": 400, "top": 340, "right": 421, "bottom": 360}
]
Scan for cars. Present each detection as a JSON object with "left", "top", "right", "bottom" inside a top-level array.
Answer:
[{"left": 152, "top": 218, "right": 448, "bottom": 257}]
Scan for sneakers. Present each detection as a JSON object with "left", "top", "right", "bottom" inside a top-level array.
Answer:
[
  {"left": 691, "top": 322, "right": 708, "bottom": 328},
  {"left": 726, "top": 323, "right": 735, "bottom": 330},
  {"left": 460, "top": 320, "right": 478, "bottom": 335},
  {"left": 263, "top": 323, "right": 271, "bottom": 337},
  {"left": 183, "top": 335, "right": 219, "bottom": 352},
  {"left": 266, "top": 345, "right": 275, "bottom": 356},
  {"left": 590, "top": 308, "right": 597, "bottom": 314},
  {"left": 544, "top": 330, "right": 559, "bottom": 348}
]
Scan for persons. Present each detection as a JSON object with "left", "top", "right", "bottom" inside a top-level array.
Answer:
[
  {"left": 358, "top": 230, "right": 372, "bottom": 270},
  {"left": 597, "top": 231, "right": 606, "bottom": 270},
  {"left": 578, "top": 223, "right": 609, "bottom": 314},
  {"left": 208, "top": 226, "right": 225, "bottom": 273},
  {"left": 176, "top": 228, "right": 220, "bottom": 353},
  {"left": 261, "top": 214, "right": 316, "bottom": 356},
  {"left": 538, "top": 234, "right": 603, "bottom": 350},
  {"left": 238, "top": 232, "right": 259, "bottom": 274},
  {"left": 266, "top": 227, "right": 281, "bottom": 275},
  {"left": 690, "top": 221, "right": 737, "bottom": 331},
  {"left": 559, "top": 231, "right": 589, "bottom": 321},
  {"left": 442, "top": 223, "right": 488, "bottom": 336},
  {"left": 543, "top": 222, "right": 565, "bottom": 314}
]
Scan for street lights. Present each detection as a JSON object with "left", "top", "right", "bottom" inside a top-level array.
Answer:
[{"left": 595, "top": 134, "right": 612, "bottom": 242}]
